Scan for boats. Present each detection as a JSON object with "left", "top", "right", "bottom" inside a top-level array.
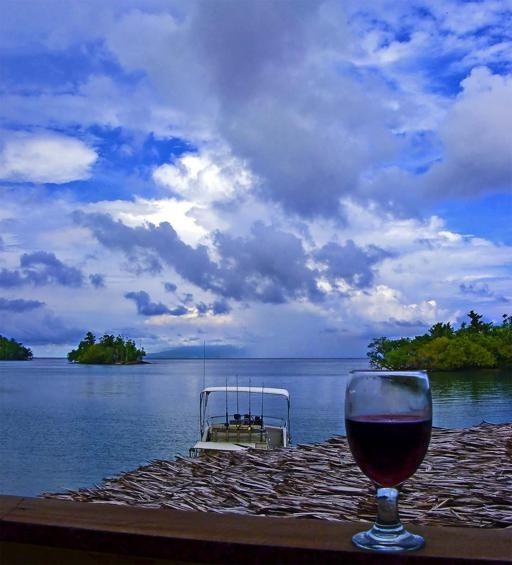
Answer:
[{"left": 188, "top": 385, "right": 291, "bottom": 456}]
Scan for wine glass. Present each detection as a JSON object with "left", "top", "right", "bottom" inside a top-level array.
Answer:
[{"left": 343, "top": 367, "right": 434, "bottom": 553}]
